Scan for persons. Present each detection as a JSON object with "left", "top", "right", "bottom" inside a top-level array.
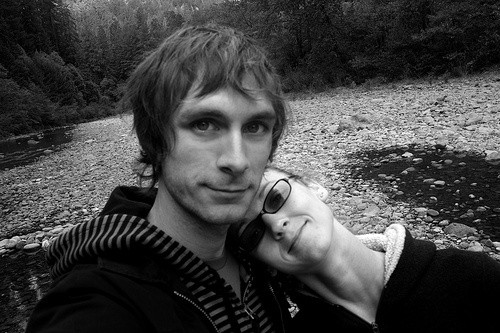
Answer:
[
  {"left": 26, "top": 24, "right": 289, "bottom": 333},
  {"left": 237, "top": 166, "right": 500, "bottom": 333}
]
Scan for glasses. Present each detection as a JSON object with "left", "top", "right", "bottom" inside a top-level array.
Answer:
[{"left": 238, "top": 175, "right": 295, "bottom": 254}]
